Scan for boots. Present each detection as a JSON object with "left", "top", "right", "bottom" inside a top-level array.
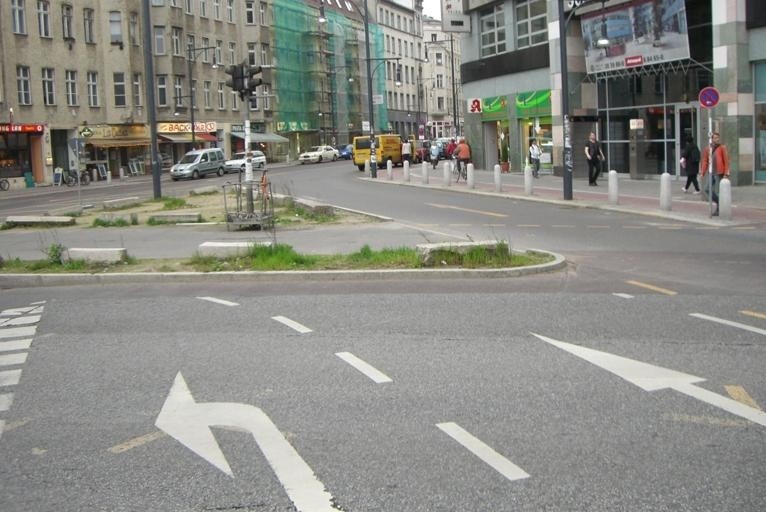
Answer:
[{"left": 224, "top": 150, "right": 266, "bottom": 174}]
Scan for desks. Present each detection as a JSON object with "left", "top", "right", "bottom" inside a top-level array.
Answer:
[
  {"left": 318, "top": 65, "right": 354, "bottom": 144},
  {"left": 365, "top": 57, "right": 401, "bottom": 178},
  {"left": 184, "top": 42, "right": 221, "bottom": 150},
  {"left": 424, "top": 39, "right": 456, "bottom": 136}
]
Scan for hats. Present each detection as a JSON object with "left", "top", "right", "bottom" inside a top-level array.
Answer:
[
  {"left": 414, "top": 137, "right": 456, "bottom": 165},
  {"left": 298, "top": 144, "right": 353, "bottom": 164}
]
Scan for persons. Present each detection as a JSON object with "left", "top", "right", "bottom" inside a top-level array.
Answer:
[
  {"left": 401, "top": 139, "right": 411, "bottom": 167},
  {"left": 680, "top": 136, "right": 701, "bottom": 194},
  {"left": 447, "top": 139, "right": 458, "bottom": 156},
  {"left": 584, "top": 131, "right": 607, "bottom": 186},
  {"left": 701, "top": 132, "right": 729, "bottom": 217},
  {"left": 452, "top": 138, "right": 470, "bottom": 173},
  {"left": 529, "top": 137, "right": 542, "bottom": 179}
]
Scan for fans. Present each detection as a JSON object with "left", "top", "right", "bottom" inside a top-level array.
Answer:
[
  {"left": 53, "top": 173, "right": 61, "bottom": 184},
  {"left": 130, "top": 163, "right": 145, "bottom": 173},
  {"left": 98, "top": 165, "right": 106, "bottom": 176}
]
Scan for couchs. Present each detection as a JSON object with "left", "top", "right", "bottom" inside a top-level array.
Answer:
[{"left": 225, "top": 58, "right": 262, "bottom": 102}]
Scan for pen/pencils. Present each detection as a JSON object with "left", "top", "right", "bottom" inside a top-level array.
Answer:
[
  {"left": 170, "top": 148, "right": 226, "bottom": 181},
  {"left": 353, "top": 134, "right": 416, "bottom": 171}
]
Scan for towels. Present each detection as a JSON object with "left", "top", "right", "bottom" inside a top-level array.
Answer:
[{"left": 24, "top": 172, "right": 33, "bottom": 188}]
